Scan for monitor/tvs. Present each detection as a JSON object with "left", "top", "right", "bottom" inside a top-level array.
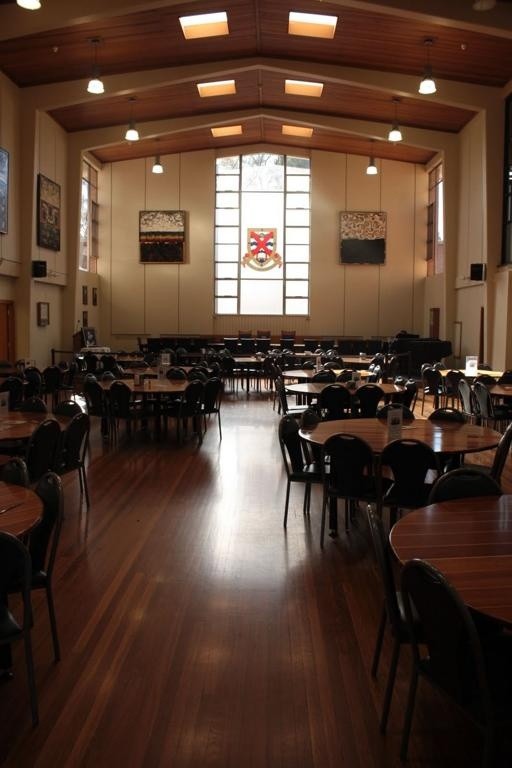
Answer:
[
  {"left": 139, "top": 242, "right": 184, "bottom": 264},
  {"left": 341, "top": 239, "right": 385, "bottom": 264}
]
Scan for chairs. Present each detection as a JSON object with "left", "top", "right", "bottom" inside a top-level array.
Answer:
[
  {"left": 363, "top": 498, "right": 413, "bottom": 743},
  {"left": 270, "top": 328, "right": 511, "bottom": 551},
  {"left": 0, "top": 327, "right": 269, "bottom": 448},
  {"left": 396, "top": 555, "right": 509, "bottom": 766},
  {"left": 0, "top": 375, "right": 99, "bottom": 725}
]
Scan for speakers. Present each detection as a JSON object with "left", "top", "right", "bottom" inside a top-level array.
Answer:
[
  {"left": 470, "top": 263, "right": 485, "bottom": 281},
  {"left": 32, "top": 260, "right": 48, "bottom": 278}
]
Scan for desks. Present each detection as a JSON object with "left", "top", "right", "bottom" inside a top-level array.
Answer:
[{"left": 386, "top": 484, "right": 510, "bottom": 637}]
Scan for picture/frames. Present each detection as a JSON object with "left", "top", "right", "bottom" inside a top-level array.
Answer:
[
  {"left": 339, "top": 210, "right": 387, "bottom": 265},
  {"left": 37, "top": 301, "right": 50, "bottom": 326},
  {"left": 139, "top": 210, "right": 186, "bottom": 264},
  {"left": 1, "top": 147, "right": 11, "bottom": 233},
  {"left": 36, "top": 174, "right": 61, "bottom": 250}
]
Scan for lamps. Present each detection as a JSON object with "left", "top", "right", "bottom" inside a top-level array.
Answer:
[
  {"left": 364, "top": 36, "right": 437, "bottom": 175},
  {"left": 18, "top": 1, "right": 164, "bottom": 175}
]
